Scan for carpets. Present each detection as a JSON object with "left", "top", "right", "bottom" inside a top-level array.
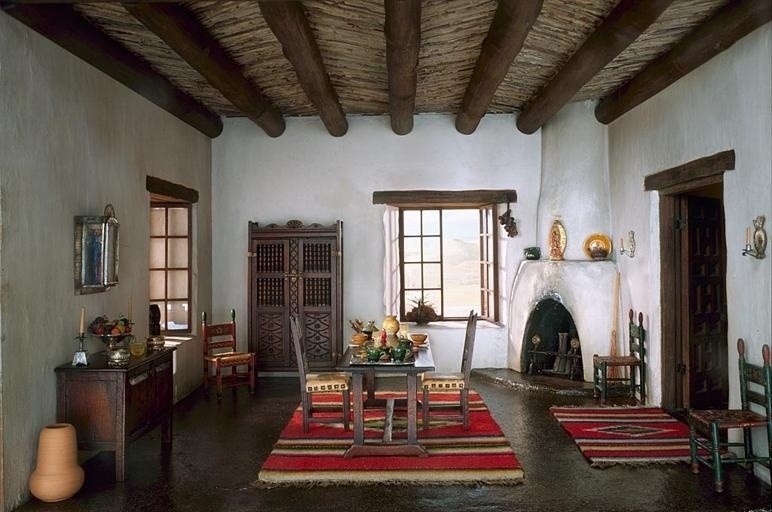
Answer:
[
  {"left": 259, "top": 386, "right": 525, "bottom": 486},
  {"left": 548, "top": 405, "right": 736, "bottom": 470}
]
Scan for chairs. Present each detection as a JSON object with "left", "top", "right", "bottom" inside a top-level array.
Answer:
[
  {"left": 593, "top": 309, "right": 646, "bottom": 405},
  {"left": 288, "top": 315, "right": 350, "bottom": 433},
  {"left": 689, "top": 339, "right": 772, "bottom": 492},
  {"left": 421, "top": 310, "right": 478, "bottom": 430},
  {"left": 202, "top": 308, "right": 254, "bottom": 400}
]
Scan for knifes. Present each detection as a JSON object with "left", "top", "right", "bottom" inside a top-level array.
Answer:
[{"left": 349, "top": 319, "right": 363, "bottom": 333}]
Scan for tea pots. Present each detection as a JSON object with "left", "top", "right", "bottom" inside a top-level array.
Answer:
[
  {"left": 379, "top": 346, "right": 394, "bottom": 359},
  {"left": 524, "top": 247, "right": 541, "bottom": 259}
]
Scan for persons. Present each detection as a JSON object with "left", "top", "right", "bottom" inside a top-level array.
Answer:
[{"left": 552, "top": 229, "right": 561, "bottom": 257}]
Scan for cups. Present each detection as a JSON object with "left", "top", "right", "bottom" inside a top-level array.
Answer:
[
  {"left": 398, "top": 339, "right": 413, "bottom": 350},
  {"left": 127, "top": 341, "right": 145, "bottom": 357},
  {"left": 391, "top": 348, "right": 410, "bottom": 363},
  {"left": 367, "top": 348, "right": 384, "bottom": 361}
]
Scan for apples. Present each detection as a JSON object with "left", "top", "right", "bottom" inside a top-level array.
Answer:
[{"left": 111, "top": 328, "right": 121, "bottom": 334}]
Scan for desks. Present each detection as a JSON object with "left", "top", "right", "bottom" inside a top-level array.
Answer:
[{"left": 54, "top": 346, "right": 178, "bottom": 481}]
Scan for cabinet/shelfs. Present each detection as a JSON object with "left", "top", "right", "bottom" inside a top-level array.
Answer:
[{"left": 247, "top": 220, "right": 343, "bottom": 377}]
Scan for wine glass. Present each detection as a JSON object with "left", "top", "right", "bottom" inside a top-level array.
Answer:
[
  {"left": 361, "top": 329, "right": 379, "bottom": 340},
  {"left": 90, "top": 331, "right": 131, "bottom": 356}
]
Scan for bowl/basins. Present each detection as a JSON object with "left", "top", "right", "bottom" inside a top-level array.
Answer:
[
  {"left": 351, "top": 335, "right": 367, "bottom": 341},
  {"left": 410, "top": 334, "right": 428, "bottom": 342}
]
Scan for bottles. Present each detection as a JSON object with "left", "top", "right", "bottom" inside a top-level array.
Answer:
[{"left": 383, "top": 315, "right": 400, "bottom": 333}]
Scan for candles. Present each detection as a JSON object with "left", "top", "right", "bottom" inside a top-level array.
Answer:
[
  {"left": 80, "top": 307, "right": 85, "bottom": 332},
  {"left": 620, "top": 238, "right": 623, "bottom": 248},
  {"left": 128, "top": 296, "right": 132, "bottom": 320},
  {"left": 746, "top": 227, "right": 751, "bottom": 244}
]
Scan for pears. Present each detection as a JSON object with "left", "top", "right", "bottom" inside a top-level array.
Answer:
[{"left": 116, "top": 323, "right": 126, "bottom": 334}]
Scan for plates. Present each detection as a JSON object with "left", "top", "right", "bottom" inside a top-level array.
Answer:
[
  {"left": 352, "top": 340, "right": 365, "bottom": 345},
  {"left": 583, "top": 233, "right": 612, "bottom": 258},
  {"left": 412, "top": 341, "right": 428, "bottom": 347}
]
